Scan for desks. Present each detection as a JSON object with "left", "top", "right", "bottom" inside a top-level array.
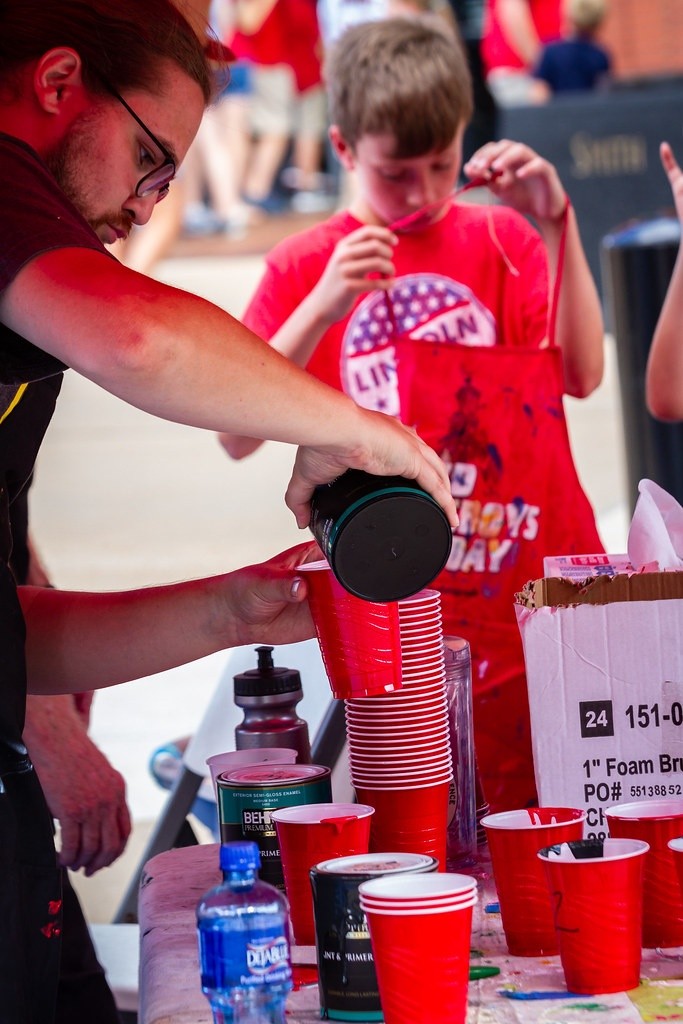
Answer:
[{"left": 139, "top": 837, "right": 682, "bottom": 1023}]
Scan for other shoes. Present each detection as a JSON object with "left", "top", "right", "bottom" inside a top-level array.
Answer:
[
  {"left": 181, "top": 197, "right": 265, "bottom": 236},
  {"left": 289, "top": 189, "right": 334, "bottom": 212}
]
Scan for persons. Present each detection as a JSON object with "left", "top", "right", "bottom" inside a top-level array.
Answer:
[
  {"left": 101, "top": 0, "right": 461, "bottom": 273},
  {"left": 21, "top": 540, "right": 133, "bottom": 877},
  {"left": 528, "top": 0, "right": 613, "bottom": 105},
  {"left": 645, "top": 141, "right": 683, "bottom": 420},
  {"left": 0, "top": 1, "right": 460, "bottom": 1024},
  {"left": 216, "top": 17, "right": 605, "bottom": 846}
]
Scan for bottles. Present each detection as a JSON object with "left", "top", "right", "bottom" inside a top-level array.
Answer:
[
  {"left": 443, "top": 637, "right": 476, "bottom": 871},
  {"left": 195, "top": 843, "right": 294, "bottom": 1024},
  {"left": 232, "top": 647, "right": 312, "bottom": 767}
]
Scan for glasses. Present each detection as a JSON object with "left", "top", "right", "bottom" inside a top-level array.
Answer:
[{"left": 82, "top": 61, "right": 176, "bottom": 201}]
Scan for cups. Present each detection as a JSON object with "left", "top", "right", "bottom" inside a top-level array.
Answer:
[
  {"left": 206, "top": 747, "right": 298, "bottom": 804},
  {"left": 307, "top": 467, "right": 452, "bottom": 602},
  {"left": 343, "top": 588, "right": 454, "bottom": 870},
  {"left": 449, "top": 772, "right": 493, "bottom": 845},
  {"left": 294, "top": 560, "right": 400, "bottom": 699},
  {"left": 268, "top": 797, "right": 683, "bottom": 1024}
]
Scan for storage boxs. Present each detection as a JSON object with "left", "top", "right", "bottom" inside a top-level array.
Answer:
[{"left": 514, "top": 571, "right": 682, "bottom": 843}]
